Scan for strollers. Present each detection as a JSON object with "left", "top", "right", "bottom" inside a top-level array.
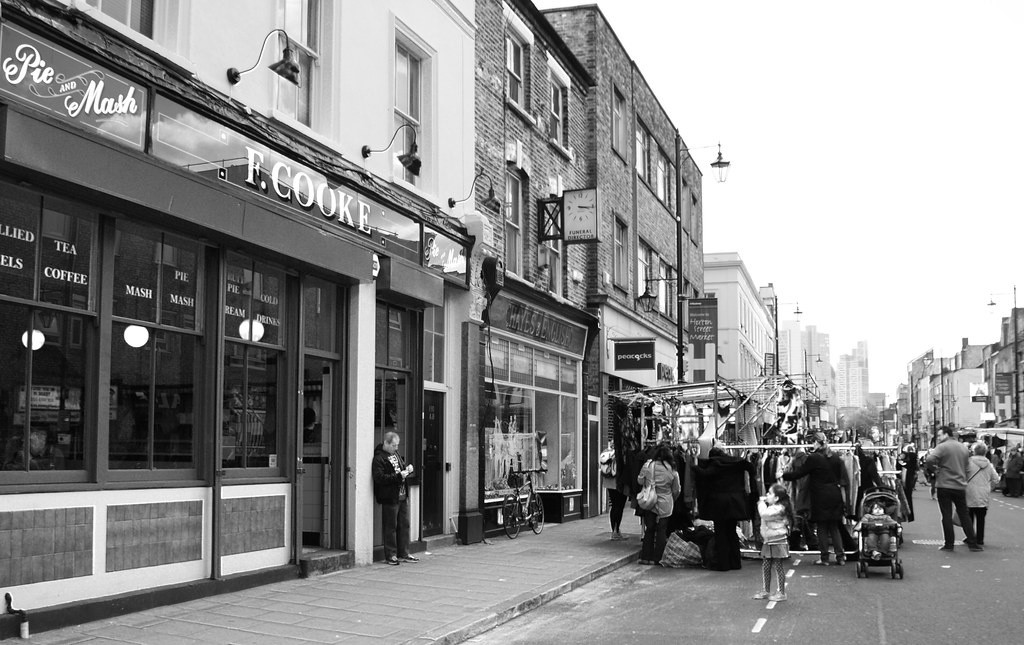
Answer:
[{"left": 854, "top": 485, "right": 905, "bottom": 580}]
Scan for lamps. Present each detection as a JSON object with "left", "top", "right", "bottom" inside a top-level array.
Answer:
[
  {"left": 123, "top": 252, "right": 150, "bottom": 348},
  {"left": 447, "top": 172, "right": 501, "bottom": 216},
  {"left": 22, "top": 329, "right": 46, "bottom": 351},
  {"left": 362, "top": 123, "right": 422, "bottom": 176},
  {"left": 238, "top": 319, "right": 266, "bottom": 341},
  {"left": 226, "top": 29, "right": 300, "bottom": 86}
]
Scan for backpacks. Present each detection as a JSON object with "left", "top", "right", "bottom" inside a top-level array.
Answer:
[{"left": 600, "top": 448, "right": 617, "bottom": 478}]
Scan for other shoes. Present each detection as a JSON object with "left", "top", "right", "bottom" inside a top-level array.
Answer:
[
  {"left": 610, "top": 532, "right": 630, "bottom": 541},
  {"left": 938, "top": 545, "right": 953, "bottom": 551},
  {"left": 768, "top": 592, "right": 787, "bottom": 602},
  {"left": 963, "top": 539, "right": 983, "bottom": 545},
  {"left": 750, "top": 591, "right": 770, "bottom": 599},
  {"left": 837, "top": 560, "right": 846, "bottom": 566},
  {"left": 874, "top": 552, "right": 881, "bottom": 560},
  {"left": 814, "top": 558, "right": 829, "bottom": 566},
  {"left": 968, "top": 542, "right": 984, "bottom": 551},
  {"left": 639, "top": 560, "right": 659, "bottom": 564}
]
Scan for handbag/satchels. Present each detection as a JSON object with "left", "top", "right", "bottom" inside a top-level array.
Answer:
[
  {"left": 659, "top": 529, "right": 704, "bottom": 568},
  {"left": 952, "top": 505, "right": 961, "bottom": 527},
  {"left": 636, "top": 482, "right": 658, "bottom": 511}
]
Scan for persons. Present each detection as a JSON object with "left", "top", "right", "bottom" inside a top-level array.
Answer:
[
  {"left": 372, "top": 431, "right": 420, "bottom": 565},
  {"left": 752, "top": 434, "right": 851, "bottom": 601},
  {"left": 599, "top": 433, "right": 682, "bottom": 568},
  {"left": 895, "top": 424, "right": 1024, "bottom": 553}
]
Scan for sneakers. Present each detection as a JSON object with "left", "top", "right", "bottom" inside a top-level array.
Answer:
[
  {"left": 386, "top": 555, "right": 399, "bottom": 565},
  {"left": 398, "top": 554, "right": 419, "bottom": 562}
]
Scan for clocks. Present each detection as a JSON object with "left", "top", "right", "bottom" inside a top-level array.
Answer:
[{"left": 564, "top": 187, "right": 602, "bottom": 243}]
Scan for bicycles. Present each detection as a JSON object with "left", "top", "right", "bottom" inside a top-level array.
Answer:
[{"left": 502, "top": 469, "right": 549, "bottom": 539}]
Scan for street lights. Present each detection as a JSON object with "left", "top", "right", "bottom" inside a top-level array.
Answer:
[
  {"left": 773, "top": 294, "right": 804, "bottom": 375},
  {"left": 987, "top": 288, "right": 1020, "bottom": 426},
  {"left": 674, "top": 127, "right": 731, "bottom": 385},
  {"left": 803, "top": 349, "right": 824, "bottom": 428}
]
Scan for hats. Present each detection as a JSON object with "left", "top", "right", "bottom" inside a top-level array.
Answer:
[{"left": 1011, "top": 447, "right": 1019, "bottom": 452}]
